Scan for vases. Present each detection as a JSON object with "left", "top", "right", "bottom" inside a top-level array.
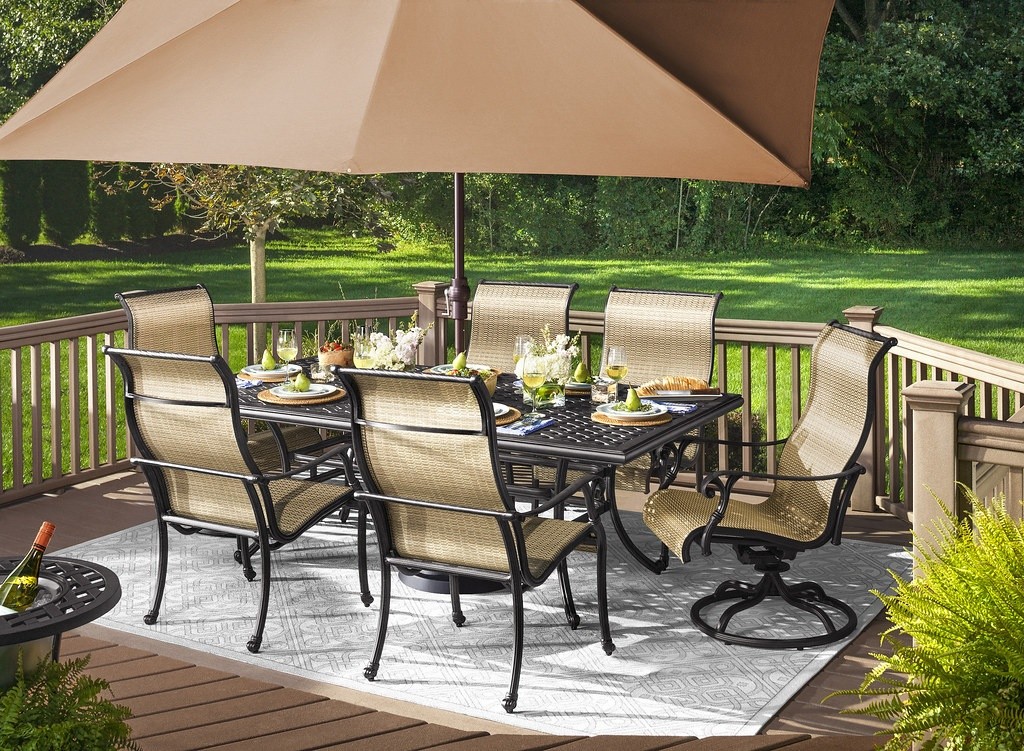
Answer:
[{"left": 522, "top": 378, "right": 565, "bottom": 408}]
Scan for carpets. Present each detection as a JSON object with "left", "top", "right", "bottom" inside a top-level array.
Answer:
[{"left": 44, "top": 465, "right": 915, "bottom": 736}]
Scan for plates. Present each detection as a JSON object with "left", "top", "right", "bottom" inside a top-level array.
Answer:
[
  {"left": 241, "top": 363, "right": 303, "bottom": 379},
  {"left": 492, "top": 402, "right": 510, "bottom": 416},
  {"left": 596, "top": 402, "right": 668, "bottom": 422},
  {"left": 625, "top": 388, "right": 723, "bottom": 402},
  {"left": 270, "top": 383, "right": 337, "bottom": 400},
  {"left": 429, "top": 364, "right": 490, "bottom": 374},
  {"left": 558, "top": 376, "right": 614, "bottom": 388}
]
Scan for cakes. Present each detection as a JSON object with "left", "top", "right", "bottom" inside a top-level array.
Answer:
[{"left": 318, "top": 340, "right": 354, "bottom": 369}]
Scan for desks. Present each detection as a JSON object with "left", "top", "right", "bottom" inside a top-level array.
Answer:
[{"left": 233, "top": 353, "right": 745, "bottom": 629}]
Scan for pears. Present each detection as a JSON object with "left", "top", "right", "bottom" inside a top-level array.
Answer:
[
  {"left": 625, "top": 382, "right": 641, "bottom": 411},
  {"left": 574, "top": 358, "right": 588, "bottom": 382},
  {"left": 262, "top": 349, "right": 276, "bottom": 370},
  {"left": 452, "top": 350, "right": 468, "bottom": 371},
  {"left": 295, "top": 370, "right": 310, "bottom": 392}
]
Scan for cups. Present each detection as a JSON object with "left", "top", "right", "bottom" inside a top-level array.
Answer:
[
  {"left": 352, "top": 338, "right": 376, "bottom": 370},
  {"left": 352, "top": 325, "right": 370, "bottom": 344},
  {"left": 310, "top": 363, "right": 331, "bottom": 382},
  {"left": 591, "top": 377, "right": 616, "bottom": 403},
  {"left": 513, "top": 335, "right": 533, "bottom": 365}
]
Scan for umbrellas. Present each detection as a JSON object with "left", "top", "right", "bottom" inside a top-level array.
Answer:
[{"left": 0, "top": 0, "right": 840, "bottom": 357}]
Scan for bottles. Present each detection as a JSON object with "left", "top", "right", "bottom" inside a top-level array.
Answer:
[{"left": 0, "top": 520, "right": 56, "bottom": 616}]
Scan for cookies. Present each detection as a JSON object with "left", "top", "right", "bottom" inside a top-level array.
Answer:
[{"left": 640, "top": 375, "right": 710, "bottom": 394}]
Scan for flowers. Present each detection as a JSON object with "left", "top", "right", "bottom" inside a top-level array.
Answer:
[
  {"left": 350, "top": 309, "right": 436, "bottom": 371},
  {"left": 514, "top": 321, "right": 583, "bottom": 400}
]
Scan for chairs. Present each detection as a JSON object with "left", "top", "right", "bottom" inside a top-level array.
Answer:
[
  {"left": 642, "top": 319, "right": 899, "bottom": 650},
  {"left": 101, "top": 279, "right": 723, "bottom": 716}
]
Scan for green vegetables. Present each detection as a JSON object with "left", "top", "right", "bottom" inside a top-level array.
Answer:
[{"left": 456, "top": 369, "right": 494, "bottom": 381}]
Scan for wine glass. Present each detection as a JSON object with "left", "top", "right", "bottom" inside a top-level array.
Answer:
[
  {"left": 607, "top": 345, "right": 628, "bottom": 404},
  {"left": 276, "top": 328, "right": 299, "bottom": 386},
  {"left": 522, "top": 355, "right": 547, "bottom": 419}
]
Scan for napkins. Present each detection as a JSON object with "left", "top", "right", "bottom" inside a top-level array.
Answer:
[
  {"left": 234, "top": 377, "right": 263, "bottom": 389},
  {"left": 496, "top": 417, "right": 556, "bottom": 436},
  {"left": 653, "top": 400, "right": 699, "bottom": 414}
]
